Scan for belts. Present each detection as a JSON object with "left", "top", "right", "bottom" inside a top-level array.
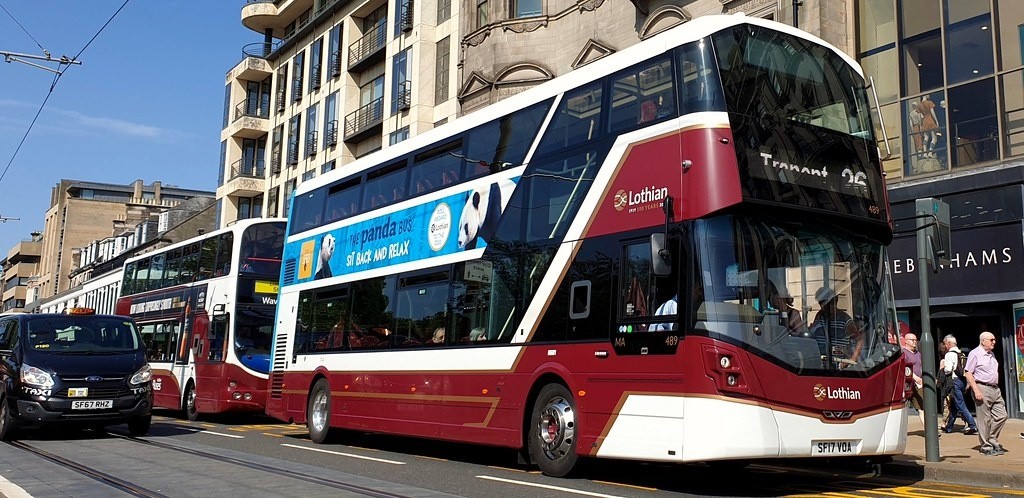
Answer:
[{"left": 976, "top": 381, "right": 998, "bottom": 388}]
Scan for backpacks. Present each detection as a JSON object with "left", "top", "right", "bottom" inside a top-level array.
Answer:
[{"left": 949, "top": 350, "right": 967, "bottom": 379}]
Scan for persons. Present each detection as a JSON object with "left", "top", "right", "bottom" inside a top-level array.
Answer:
[
  {"left": 648, "top": 277, "right": 702, "bottom": 332},
  {"left": 936, "top": 335, "right": 978, "bottom": 435},
  {"left": 469, "top": 328, "right": 487, "bottom": 341},
  {"left": 810, "top": 286, "right": 863, "bottom": 369},
  {"left": 199, "top": 266, "right": 207, "bottom": 280},
  {"left": 142, "top": 333, "right": 178, "bottom": 361},
  {"left": 964, "top": 332, "right": 1007, "bottom": 456},
  {"left": 903, "top": 334, "right": 942, "bottom": 436},
  {"left": 908, "top": 95, "right": 946, "bottom": 166},
  {"left": 218, "top": 248, "right": 232, "bottom": 273},
  {"left": 766, "top": 284, "right": 804, "bottom": 337},
  {"left": 433, "top": 328, "right": 445, "bottom": 344}
]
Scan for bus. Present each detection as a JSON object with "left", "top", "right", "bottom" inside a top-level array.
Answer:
[
  {"left": 264, "top": 11, "right": 945, "bottom": 481},
  {"left": 110, "top": 216, "right": 289, "bottom": 421}
]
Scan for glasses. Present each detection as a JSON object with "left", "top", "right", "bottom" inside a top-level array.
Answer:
[
  {"left": 986, "top": 338, "right": 996, "bottom": 342},
  {"left": 909, "top": 339, "right": 917, "bottom": 342}
]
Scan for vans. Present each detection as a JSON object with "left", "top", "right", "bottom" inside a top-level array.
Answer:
[{"left": 0, "top": 308, "right": 159, "bottom": 442}]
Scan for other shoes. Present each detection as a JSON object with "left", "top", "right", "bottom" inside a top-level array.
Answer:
[
  {"left": 1020, "top": 432, "right": 1024, "bottom": 438},
  {"left": 994, "top": 444, "right": 1008, "bottom": 452},
  {"left": 960, "top": 426, "right": 978, "bottom": 434},
  {"left": 941, "top": 427, "right": 947, "bottom": 432},
  {"left": 979, "top": 449, "right": 998, "bottom": 456}
]
